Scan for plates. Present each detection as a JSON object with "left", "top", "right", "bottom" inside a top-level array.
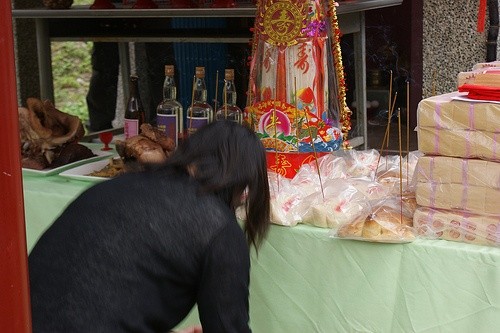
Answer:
[
  {"left": 58, "top": 154, "right": 125, "bottom": 182},
  {"left": 21, "top": 150, "right": 114, "bottom": 177}
]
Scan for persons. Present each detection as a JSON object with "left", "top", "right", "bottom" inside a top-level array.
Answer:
[{"left": 27, "top": 120, "right": 269, "bottom": 333}]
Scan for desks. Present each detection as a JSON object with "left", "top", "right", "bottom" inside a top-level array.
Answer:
[{"left": 23, "top": 142, "right": 500, "bottom": 333}]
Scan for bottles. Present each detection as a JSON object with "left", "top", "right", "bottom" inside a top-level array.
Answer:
[
  {"left": 124, "top": 74, "right": 144, "bottom": 141},
  {"left": 215, "top": 69, "right": 244, "bottom": 125},
  {"left": 187, "top": 66, "right": 214, "bottom": 137},
  {"left": 156, "top": 65, "right": 184, "bottom": 150}
]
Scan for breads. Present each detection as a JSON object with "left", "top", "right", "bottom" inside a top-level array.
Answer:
[{"left": 270, "top": 154, "right": 420, "bottom": 242}]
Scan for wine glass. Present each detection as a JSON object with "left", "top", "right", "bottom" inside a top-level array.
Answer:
[{"left": 98, "top": 132, "right": 113, "bottom": 151}]
